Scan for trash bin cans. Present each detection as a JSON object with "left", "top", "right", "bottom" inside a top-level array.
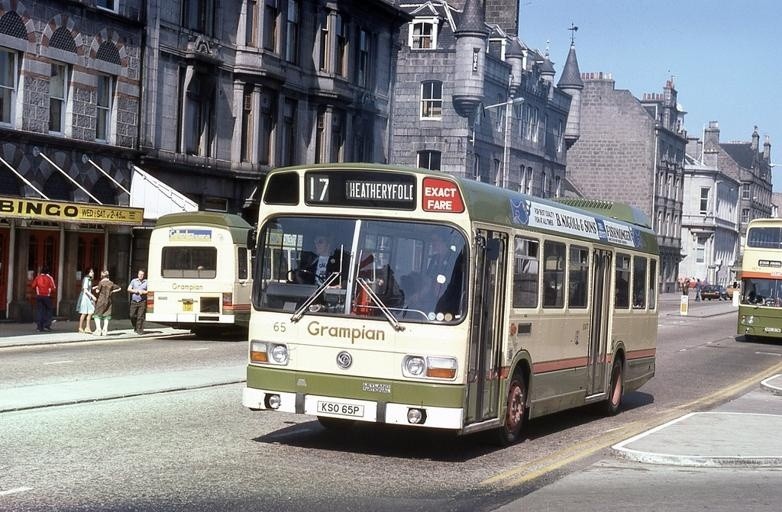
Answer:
[{"left": 665, "top": 282, "right": 676, "bottom": 293}]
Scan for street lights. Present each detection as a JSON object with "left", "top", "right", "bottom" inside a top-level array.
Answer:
[{"left": 473, "top": 97, "right": 525, "bottom": 147}]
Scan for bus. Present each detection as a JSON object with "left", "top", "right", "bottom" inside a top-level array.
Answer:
[{"left": 737, "top": 218, "right": 782, "bottom": 342}]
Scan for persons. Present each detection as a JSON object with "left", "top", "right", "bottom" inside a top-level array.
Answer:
[
  {"left": 614, "top": 270, "right": 628, "bottom": 307},
  {"left": 31, "top": 265, "right": 56, "bottom": 333},
  {"left": 292, "top": 233, "right": 346, "bottom": 289},
  {"left": 750, "top": 283, "right": 766, "bottom": 303},
  {"left": 411, "top": 228, "right": 461, "bottom": 320},
  {"left": 127, "top": 268, "right": 147, "bottom": 335},
  {"left": 75, "top": 267, "right": 121, "bottom": 336},
  {"left": 679, "top": 277, "right": 703, "bottom": 302}
]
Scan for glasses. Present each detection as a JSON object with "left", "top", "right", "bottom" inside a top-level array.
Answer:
[{"left": 314, "top": 239, "right": 325, "bottom": 245}]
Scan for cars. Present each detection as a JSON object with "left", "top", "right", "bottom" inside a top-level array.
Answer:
[{"left": 683, "top": 282, "right": 742, "bottom": 301}]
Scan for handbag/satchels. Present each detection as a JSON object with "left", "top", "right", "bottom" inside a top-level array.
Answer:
[{"left": 90, "top": 297, "right": 97, "bottom": 307}]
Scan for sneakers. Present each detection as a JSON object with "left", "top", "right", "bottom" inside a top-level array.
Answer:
[
  {"left": 79, "top": 327, "right": 107, "bottom": 336},
  {"left": 36, "top": 325, "right": 53, "bottom": 332},
  {"left": 134, "top": 328, "right": 143, "bottom": 335}
]
